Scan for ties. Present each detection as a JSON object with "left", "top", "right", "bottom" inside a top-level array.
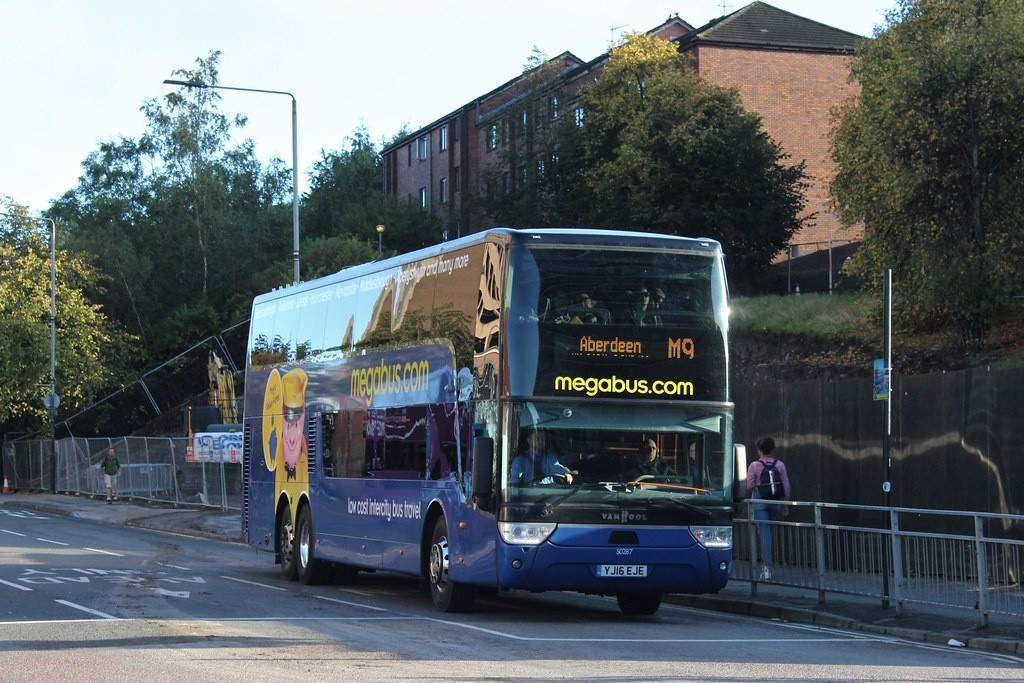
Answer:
[{"left": 533, "top": 454, "right": 541, "bottom": 476}]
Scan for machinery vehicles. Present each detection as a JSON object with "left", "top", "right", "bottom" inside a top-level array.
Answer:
[{"left": 181, "top": 348, "right": 244, "bottom": 475}]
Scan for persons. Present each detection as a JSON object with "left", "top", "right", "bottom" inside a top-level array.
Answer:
[
  {"left": 746, "top": 437, "right": 791, "bottom": 580},
  {"left": 100, "top": 448, "right": 122, "bottom": 503},
  {"left": 509, "top": 288, "right": 680, "bottom": 486}
]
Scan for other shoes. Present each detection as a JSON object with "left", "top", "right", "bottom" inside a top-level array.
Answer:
[
  {"left": 107, "top": 498, "right": 113, "bottom": 504},
  {"left": 759, "top": 570, "right": 771, "bottom": 579}
]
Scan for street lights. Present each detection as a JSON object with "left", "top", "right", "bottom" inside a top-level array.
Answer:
[
  {"left": 162, "top": 80, "right": 301, "bottom": 285},
  {"left": 1, "top": 212, "right": 56, "bottom": 495},
  {"left": 376, "top": 225, "right": 385, "bottom": 261}
]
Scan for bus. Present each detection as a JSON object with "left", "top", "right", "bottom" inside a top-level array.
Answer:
[{"left": 237, "top": 224, "right": 748, "bottom": 618}]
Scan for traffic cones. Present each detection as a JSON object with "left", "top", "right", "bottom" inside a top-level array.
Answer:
[{"left": 2, "top": 476, "right": 9, "bottom": 495}]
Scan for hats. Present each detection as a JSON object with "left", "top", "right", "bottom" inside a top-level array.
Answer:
[{"left": 757, "top": 437, "right": 775, "bottom": 454}]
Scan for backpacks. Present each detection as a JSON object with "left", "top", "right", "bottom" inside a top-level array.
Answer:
[{"left": 756, "top": 458, "right": 785, "bottom": 499}]
